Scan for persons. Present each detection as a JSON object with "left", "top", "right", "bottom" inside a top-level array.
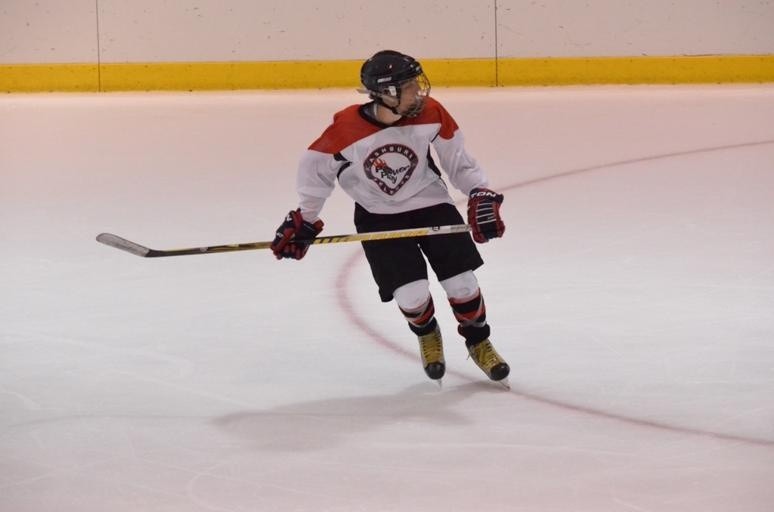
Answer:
[{"left": 269, "top": 51, "right": 510, "bottom": 380}]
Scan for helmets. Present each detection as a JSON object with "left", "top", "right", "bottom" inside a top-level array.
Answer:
[{"left": 361, "top": 50, "right": 431, "bottom": 119}]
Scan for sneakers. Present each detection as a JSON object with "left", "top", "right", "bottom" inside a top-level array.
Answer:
[
  {"left": 466, "top": 339, "right": 509, "bottom": 380},
  {"left": 418, "top": 324, "right": 445, "bottom": 379}
]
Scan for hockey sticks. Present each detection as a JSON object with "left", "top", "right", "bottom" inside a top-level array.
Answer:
[{"left": 95, "top": 225, "right": 471, "bottom": 257}]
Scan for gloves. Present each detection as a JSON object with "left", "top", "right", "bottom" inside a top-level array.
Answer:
[
  {"left": 272, "top": 208, "right": 324, "bottom": 258},
  {"left": 467, "top": 188, "right": 504, "bottom": 243}
]
